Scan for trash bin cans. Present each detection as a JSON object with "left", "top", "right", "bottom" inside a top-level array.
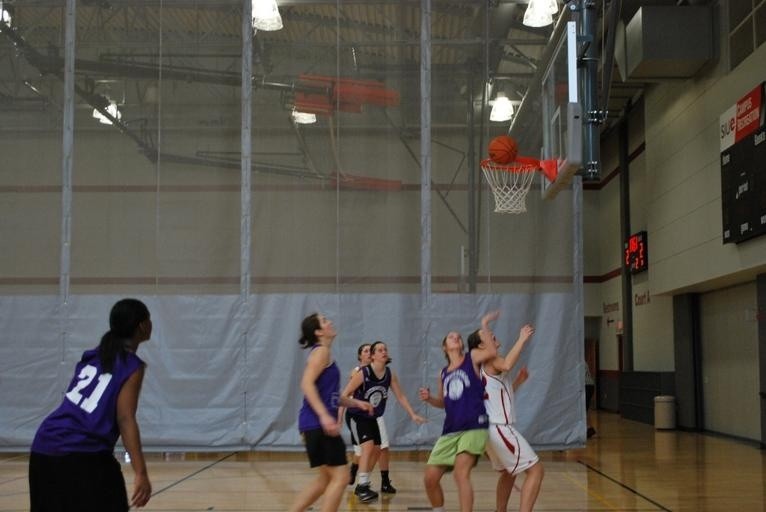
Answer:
[{"left": 654, "top": 396, "right": 676, "bottom": 430}]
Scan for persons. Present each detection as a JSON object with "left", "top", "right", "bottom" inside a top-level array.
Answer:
[
  {"left": 417, "top": 308, "right": 502, "bottom": 512},
  {"left": 338, "top": 340, "right": 427, "bottom": 505},
  {"left": 289, "top": 311, "right": 349, "bottom": 512},
  {"left": 584, "top": 357, "right": 600, "bottom": 441},
  {"left": 25, "top": 298, "right": 154, "bottom": 512},
  {"left": 337, "top": 342, "right": 397, "bottom": 494},
  {"left": 467, "top": 320, "right": 547, "bottom": 512}
]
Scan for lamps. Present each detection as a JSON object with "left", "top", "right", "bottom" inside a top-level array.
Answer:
[
  {"left": 521, "top": 0, "right": 560, "bottom": 28},
  {"left": 243, "top": 0, "right": 287, "bottom": 34},
  {"left": 92, "top": 96, "right": 126, "bottom": 128},
  {"left": 487, "top": 93, "right": 516, "bottom": 124}
]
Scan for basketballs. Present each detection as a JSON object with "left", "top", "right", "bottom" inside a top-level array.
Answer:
[{"left": 487, "top": 135, "right": 518, "bottom": 164}]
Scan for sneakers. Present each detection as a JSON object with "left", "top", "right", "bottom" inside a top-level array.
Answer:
[
  {"left": 353, "top": 485, "right": 381, "bottom": 504},
  {"left": 379, "top": 479, "right": 398, "bottom": 495}
]
